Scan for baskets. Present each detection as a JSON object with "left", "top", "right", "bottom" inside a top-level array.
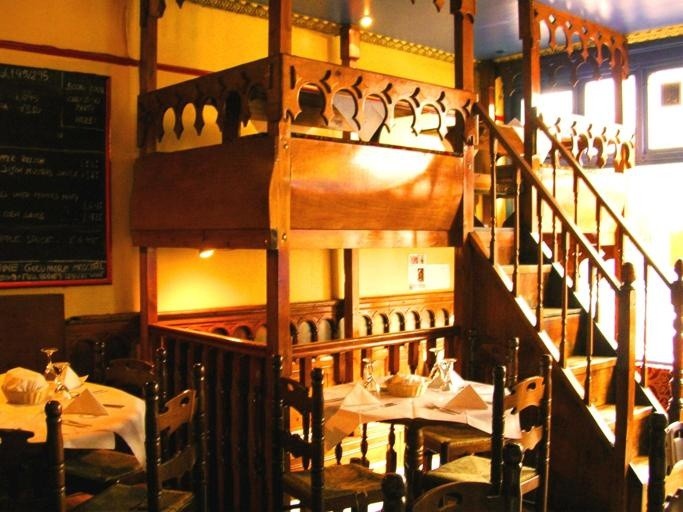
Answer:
[
  {"left": 1, "top": 383, "right": 49, "bottom": 405},
  {"left": 384, "top": 376, "right": 431, "bottom": 398}
]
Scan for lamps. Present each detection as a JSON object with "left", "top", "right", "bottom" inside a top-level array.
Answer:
[{"left": 199, "top": 248, "right": 216, "bottom": 258}]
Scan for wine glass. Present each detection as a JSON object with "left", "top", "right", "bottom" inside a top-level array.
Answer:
[
  {"left": 362, "top": 358, "right": 381, "bottom": 401},
  {"left": 427, "top": 347, "right": 458, "bottom": 394},
  {"left": 40, "top": 346, "right": 72, "bottom": 405}
]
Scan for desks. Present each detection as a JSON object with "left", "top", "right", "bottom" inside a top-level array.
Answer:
[{"left": 311, "top": 376, "right": 520, "bottom": 512}]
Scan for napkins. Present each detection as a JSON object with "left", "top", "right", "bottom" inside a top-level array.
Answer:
[
  {"left": 442, "top": 384, "right": 485, "bottom": 414},
  {"left": 341, "top": 380, "right": 381, "bottom": 409}
]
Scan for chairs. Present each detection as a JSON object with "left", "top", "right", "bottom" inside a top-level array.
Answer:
[
  {"left": 647, "top": 412, "right": 683, "bottom": 511},
  {"left": 422, "top": 356, "right": 552, "bottom": 508},
  {"left": 273, "top": 355, "right": 397, "bottom": 510},
  {"left": 414, "top": 331, "right": 519, "bottom": 472},
  {"left": 0, "top": 342, "right": 208, "bottom": 512}
]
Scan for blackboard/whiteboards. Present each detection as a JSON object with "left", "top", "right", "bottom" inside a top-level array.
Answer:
[{"left": 0, "top": 63, "right": 112, "bottom": 288}]
